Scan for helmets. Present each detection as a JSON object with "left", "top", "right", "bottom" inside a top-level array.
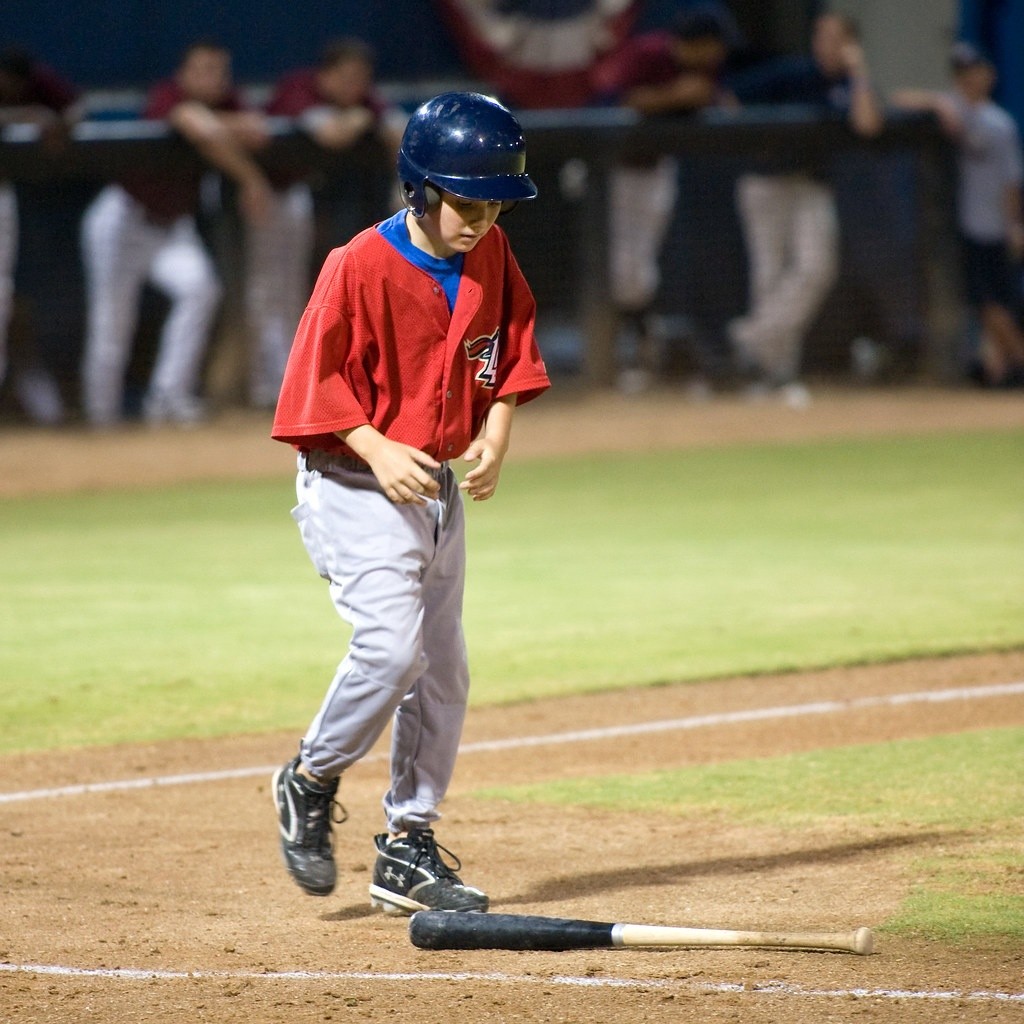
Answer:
[{"left": 395, "top": 90, "right": 539, "bottom": 219}]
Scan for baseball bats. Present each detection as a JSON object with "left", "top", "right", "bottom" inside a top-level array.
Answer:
[{"left": 407, "top": 910, "right": 874, "bottom": 956}]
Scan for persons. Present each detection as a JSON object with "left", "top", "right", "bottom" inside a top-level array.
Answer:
[
  {"left": 606, "top": 14, "right": 737, "bottom": 310},
  {"left": 890, "top": 40, "right": 1024, "bottom": 390},
  {"left": 240, "top": 37, "right": 412, "bottom": 407},
  {"left": 1, "top": 40, "right": 84, "bottom": 422},
  {"left": 725, "top": 12, "right": 887, "bottom": 399},
  {"left": 83, "top": 33, "right": 270, "bottom": 429},
  {"left": 271, "top": 91, "right": 550, "bottom": 913}
]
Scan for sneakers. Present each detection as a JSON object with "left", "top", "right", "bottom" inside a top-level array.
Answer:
[
  {"left": 368, "top": 822, "right": 490, "bottom": 920},
  {"left": 272, "top": 756, "right": 350, "bottom": 898}
]
